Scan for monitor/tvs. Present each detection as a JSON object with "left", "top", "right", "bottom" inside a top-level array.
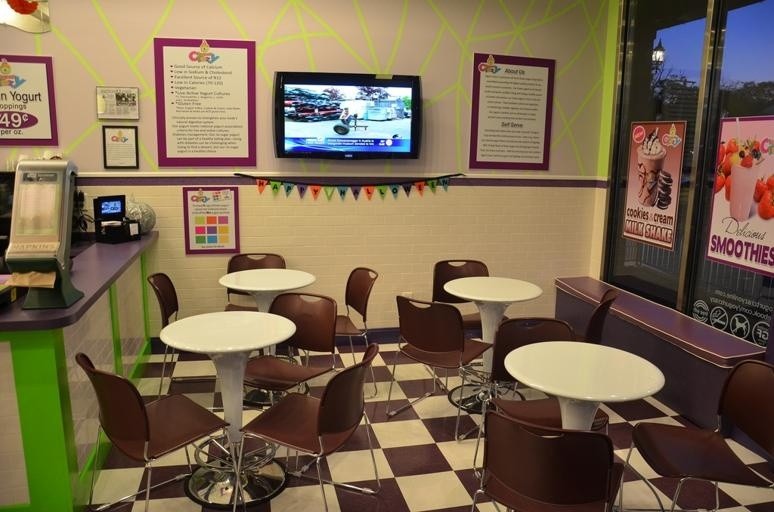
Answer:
[{"left": 272, "top": 71, "right": 424, "bottom": 159}]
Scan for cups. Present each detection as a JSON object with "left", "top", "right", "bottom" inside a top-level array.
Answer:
[
  {"left": 636, "top": 148, "right": 667, "bottom": 209},
  {"left": 730, "top": 164, "right": 759, "bottom": 223}
]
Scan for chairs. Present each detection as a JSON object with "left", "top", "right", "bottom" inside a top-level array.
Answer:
[
  {"left": 75, "top": 351, "right": 244, "bottom": 512},
  {"left": 433, "top": 258, "right": 507, "bottom": 382},
  {"left": 618, "top": 358, "right": 773, "bottom": 512},
  {"left": 471, "top": 410, "right": 625, "bottom": 512},
  {"left": 224, "top": 253, "right": 287, "bottom": 312},
  {"left": 147, "top": 273, "right": 179, "bottom": 396},
  {"left": 233, "top": 341, "right": 382, "bottom": 511},
  {"left": 474, "top": 317, "right": 609, "bottom": 483},
  {"left": 335, "top": 266, "right": 379, "bottom": 398},
  {"left": 385, "top": 294, "right": 479, "bottom": 442},
  {"left": 244, "top": 292, "right": 337, "bottom": 406}
]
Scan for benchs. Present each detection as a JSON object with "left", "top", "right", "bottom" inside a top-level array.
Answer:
[{"left": 555, "top": 275, "right": 768, "bottom": 431}]
